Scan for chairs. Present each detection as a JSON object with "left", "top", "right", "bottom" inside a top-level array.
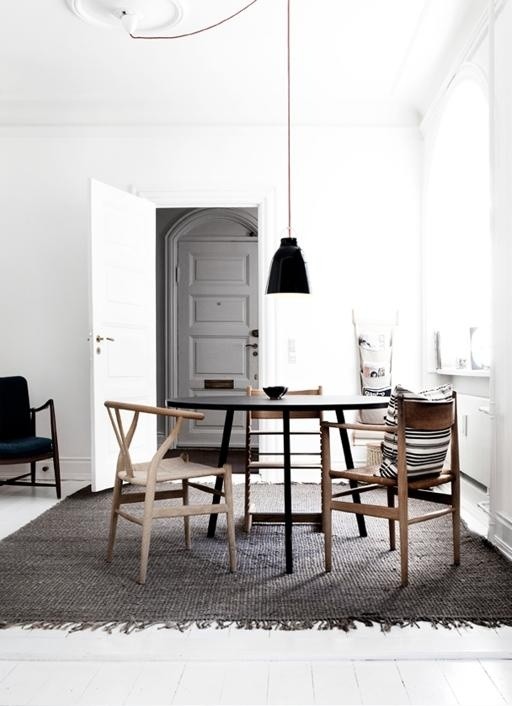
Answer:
[
  {"left": 320, "top": 399, "right": 464, "bottom": 586},
  {"left": 0, "top": 376, "right": 63, "bottom": 500},
  {"left": 107, "top": 403, "right": 236, "bottom": 585},
  {"left": 244, "top": 386, "right": 329, "bottom": 532}
]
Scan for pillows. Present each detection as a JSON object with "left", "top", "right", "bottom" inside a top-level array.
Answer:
[
  {"left": 375, "top": 385, "right": 454, "bottom": 480},
  {"left": 358, "top": 337, "right": 393, "bottom": 424}
]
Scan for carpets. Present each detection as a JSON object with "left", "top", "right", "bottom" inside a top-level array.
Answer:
[{"left": 2, "top": 486, "right": 511, "bottom": 631}]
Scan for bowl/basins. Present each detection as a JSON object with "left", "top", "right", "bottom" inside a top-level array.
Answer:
[{"left": 263, "top": 386, "right": 286, "bottom": 401}]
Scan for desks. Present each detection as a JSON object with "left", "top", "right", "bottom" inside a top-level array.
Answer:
[{"left": 165, "top": 393, "right": 391, "bottom": 576}]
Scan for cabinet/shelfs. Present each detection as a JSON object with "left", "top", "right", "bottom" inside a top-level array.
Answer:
[{"left": 439, "top": 369, "right": 488, "bottom": 490}]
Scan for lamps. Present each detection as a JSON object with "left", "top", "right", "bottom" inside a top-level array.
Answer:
[{"left": 266, "top": 4, "right": 310, "bottom": 295}]
